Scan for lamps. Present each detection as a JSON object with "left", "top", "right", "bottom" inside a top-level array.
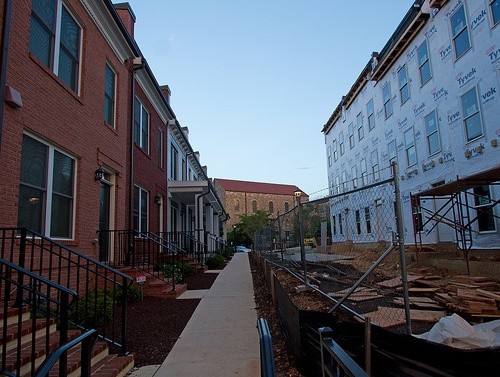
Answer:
[
  {"left": 155, "top": 192, "right": 161, "bottom": 205},
  {"left": 95, "top": 166, "right": 104, "bottom": 180}
]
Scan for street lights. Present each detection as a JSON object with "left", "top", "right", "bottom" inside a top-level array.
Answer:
[
  {"left": 294, "top": 186, "right": 308, "bottom": 286},
  {"left": 232, "top": 224, "right": 237, "bottom": 254}
]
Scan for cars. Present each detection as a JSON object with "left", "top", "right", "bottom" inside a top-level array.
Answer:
[{"left": 236, "top": 245, "right": 252, "bottom": 252}]
